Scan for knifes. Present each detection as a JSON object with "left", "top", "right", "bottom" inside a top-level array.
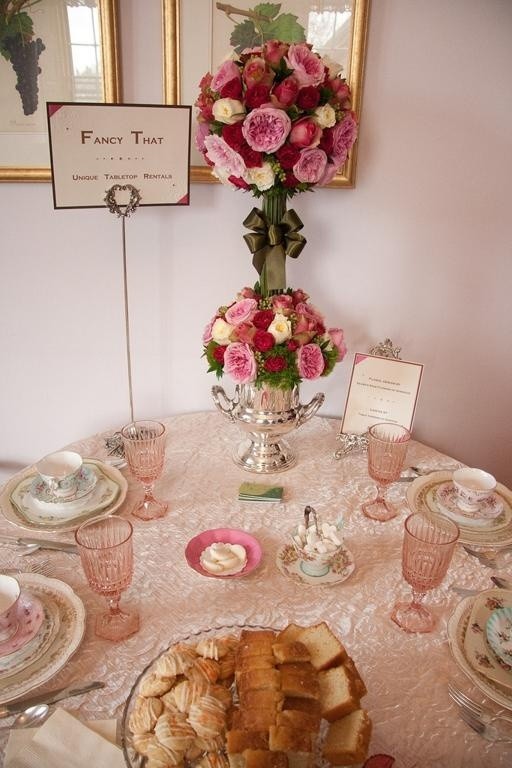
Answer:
[
  {"left": 0, "top": 534, "right": 78, "bottom": 555},
  {"left": 0, "top": 680, "right": 105, "bottom": 721}
]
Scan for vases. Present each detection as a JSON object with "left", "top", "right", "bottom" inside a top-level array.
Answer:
[{"left": 211, "top": 380, "right": 326, "bottom": 473}]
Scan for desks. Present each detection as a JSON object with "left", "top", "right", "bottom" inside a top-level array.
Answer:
[{"left": 0, "top": 410, "right": 512, "bottom": 767}]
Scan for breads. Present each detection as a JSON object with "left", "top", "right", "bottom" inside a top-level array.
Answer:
[
  {"left": 226, "top": 620, "right": 372, "bottom": 768},
  {"left": 130, "top": 632, "right": 239, "bottom": 768}
]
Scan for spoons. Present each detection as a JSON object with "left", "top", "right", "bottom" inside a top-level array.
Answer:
[
  {"left": 0, "top": 542, "right": 41, "bottom": 555},
  {"left": 492, "top": 576, "right": 512, "bottom": 588},
  {"left": 409, "top": 466, "right": 456, "bottom": 476},
  {"left": 0, "top": 704, "right": 49, "bottom": 733}
]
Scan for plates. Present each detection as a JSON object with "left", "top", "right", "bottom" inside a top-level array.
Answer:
[
  {"left": 407, "top": 471, "right": 512, "bottom": 547},
  {"left": 0, "top": 572, "right": 87, "bottom": 704},
  {"left": 276, "top": 540, "right": 356, "bottom": 589},
  {"left": 447, "top": 588, "right": 512, "bottom": 712},
  {"left": 185, "top": 528, "right": 263, "bottom": 580},
  {"left": 1, "top": 458, "right": 128, "bottom": 534},
  {"left": 120, "top": 625, "right": 331, "bottom": 767}
]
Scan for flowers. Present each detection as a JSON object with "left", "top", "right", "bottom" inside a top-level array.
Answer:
[
  {"left": 202, "top": 282, "right": 347, "bottom": 391},
  {"left": 194, "top": 39, "right": 359, "bottom": 198}
]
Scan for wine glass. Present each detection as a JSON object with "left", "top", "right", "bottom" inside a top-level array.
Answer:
[
  {"left": 362, "top": 422, "right": 411, "bottom": 520},
  {"left": 77, "top": 514, "right": 141, "bottom": 641},
  {"left": 391, "top": 509, "right": 460, "bottom": 635},
  {"left": 121, "top": 419, "right": 167, "bottom": 521}
]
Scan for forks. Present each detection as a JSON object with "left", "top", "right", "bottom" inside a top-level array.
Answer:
[
  {"left": 447, "top": 682, "right": 512, "bottom": 743},
  {"left": 462, "top": 544, "right": 512, "bottom": 570}
]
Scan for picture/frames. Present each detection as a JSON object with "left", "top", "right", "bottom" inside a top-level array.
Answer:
[
  {"left": 0, "top": 0, "right": 121, "bottom": 184},
  {"left": 160, "top": 0, "right": 373, "bottom": 191}
]
